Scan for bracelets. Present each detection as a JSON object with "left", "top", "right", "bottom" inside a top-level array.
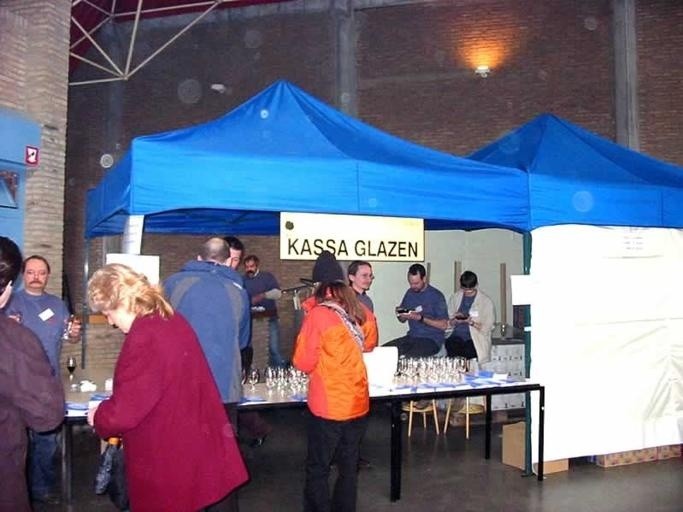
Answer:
[
  {"left": 471, "top": 321, "right": 474, "bottom": 326},
  {"left": 418, "top": 316, "right": 425, "bottom": 322}
]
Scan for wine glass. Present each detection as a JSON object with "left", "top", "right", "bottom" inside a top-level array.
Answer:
[
  {"left": 62, "top": 314, "right": 79, "bottom": 341},
  {"left": 500, "top": 323, "right": 508, "bottom": 341},
  {"left": 241, "top": 365, "right": 307, "bottom": 399},
  {"left": 66, "top": 357, "right": 77, "bottom": 379},
  {"left": 398, "top": 355, "right": 469, "bottom": 385}
]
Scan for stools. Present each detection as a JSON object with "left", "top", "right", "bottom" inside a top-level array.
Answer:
[
  {"left": 402, "top": 400, "right": 440, "bottom": 437},
  {"left": 443, "top": 358, "right": 478, "bottom": 439}
]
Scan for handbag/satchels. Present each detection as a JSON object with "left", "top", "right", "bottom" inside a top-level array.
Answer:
[{"left": 94, "top": 437, "right": 129, "bottom": 512}]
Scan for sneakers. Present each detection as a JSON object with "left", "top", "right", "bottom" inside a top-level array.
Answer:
[
  {"left": 35, "top": 492, "right": 62, "bottom": 506},
  {"left": 357, "top": 450, "right": 377, "bottom": 470}
]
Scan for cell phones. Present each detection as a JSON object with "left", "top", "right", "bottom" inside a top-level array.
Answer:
[
  {"left": 397, "top": 308, "right": 409, "bottom": 313},
  {"left": 456, "top": 315, "right": 467, "bottom": 320}
]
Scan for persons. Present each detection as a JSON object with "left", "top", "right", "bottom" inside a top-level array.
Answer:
[
  {"left": 444, "top": 270, "right": 494, "bottom": 364},
  {"left": 289, "top": 250, "right": 378, "bottom": 511},
  {"left": 0, "top": 235, "right": 66, "bottom": 512},
  {"left": 222, "top": 238, "right": 275, "bottom": 448},
  {"left": 328, "top": 260, "right": 372, "bottom": 470},
  {"left": 160, "top": 236, "right": 253, "bottom": 511},
  {"left": 86, "top": 263, "right": 249, "bottom": 512},
  {"left": 382, "top": 263, "right": 450, "bottom": 410},
  {"left": 240, "top": 254, "right": 291, "bottom": 370},
  {"left": 2, "top": 255, "right": 82, "bottom": 507}
]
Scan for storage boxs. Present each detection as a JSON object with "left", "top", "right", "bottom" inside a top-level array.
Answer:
[{"left": 502, "top": 421, "right": 569, "bottom": 474}]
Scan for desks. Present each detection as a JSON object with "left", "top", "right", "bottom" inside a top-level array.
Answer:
[{"left": 60, "top": 371, "right": 545, "bottom": 502}]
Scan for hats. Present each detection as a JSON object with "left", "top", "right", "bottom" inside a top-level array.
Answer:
[{"left": 312, "top": 251, "right": 346, "bottom": 285}]
